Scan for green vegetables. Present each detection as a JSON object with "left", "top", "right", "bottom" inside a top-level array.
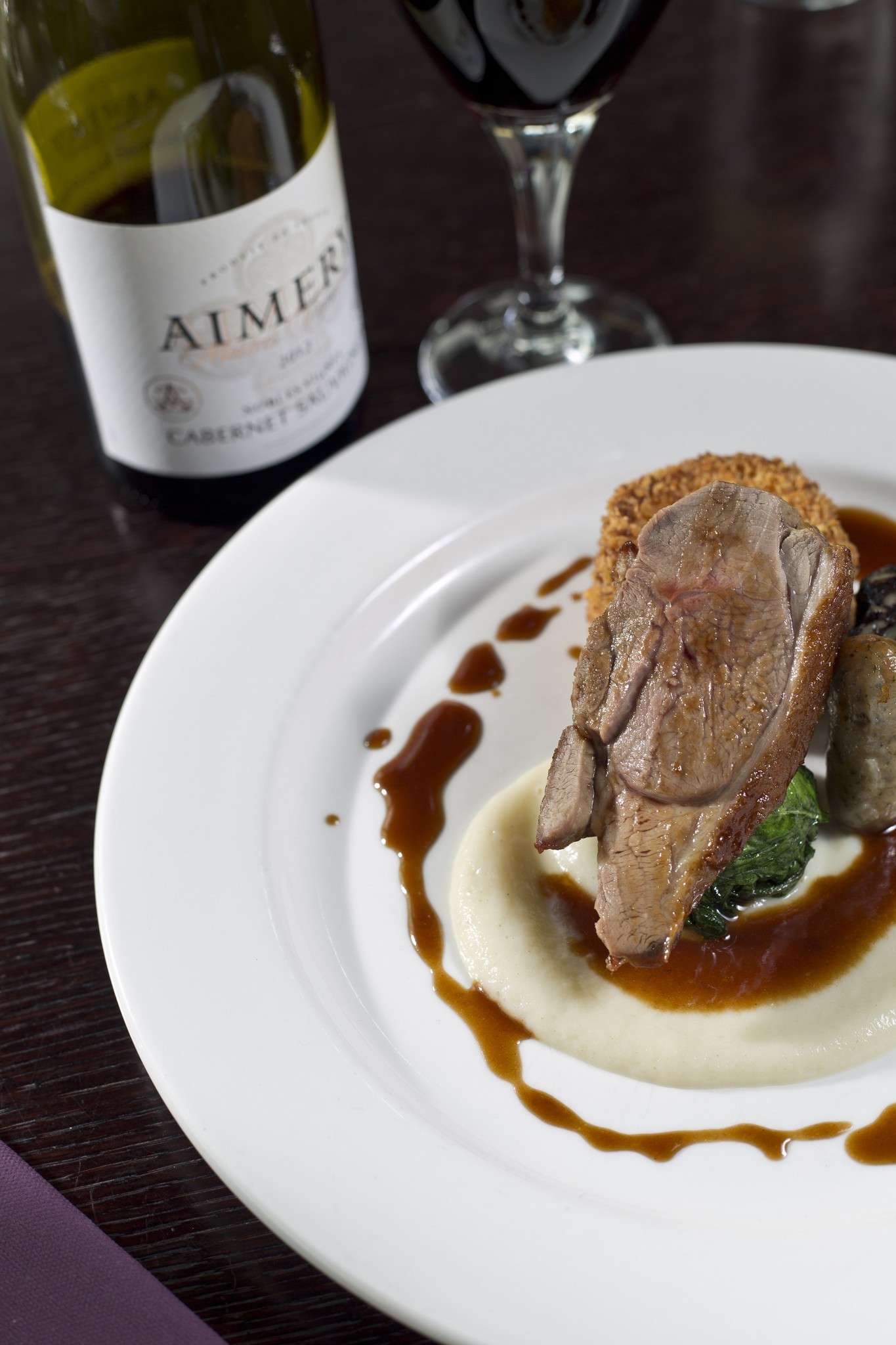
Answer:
[{"left": 686, "top": 766, "right": 832, "bottom": 940}]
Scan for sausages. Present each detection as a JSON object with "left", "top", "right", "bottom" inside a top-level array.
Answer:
[{"left": 824, "top": 636, "right": 896, "bottom": 831}]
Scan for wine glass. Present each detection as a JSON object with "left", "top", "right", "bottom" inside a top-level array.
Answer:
[{"left": 396, "top": 1, "right": 671, "bottom": 404}]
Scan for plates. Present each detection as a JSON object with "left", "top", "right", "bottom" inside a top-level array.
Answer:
[{"left": 93, "top": 345, "right": 896, "bottom": 1345}]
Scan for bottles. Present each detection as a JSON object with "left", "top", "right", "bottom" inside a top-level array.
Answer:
[{"left": 0, "top": 1, "right": 371, "bottom": 528}]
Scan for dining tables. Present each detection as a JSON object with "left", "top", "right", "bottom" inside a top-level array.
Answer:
[{"left": 0, "top": 0, "right": 896, "bottom": 1345}]
